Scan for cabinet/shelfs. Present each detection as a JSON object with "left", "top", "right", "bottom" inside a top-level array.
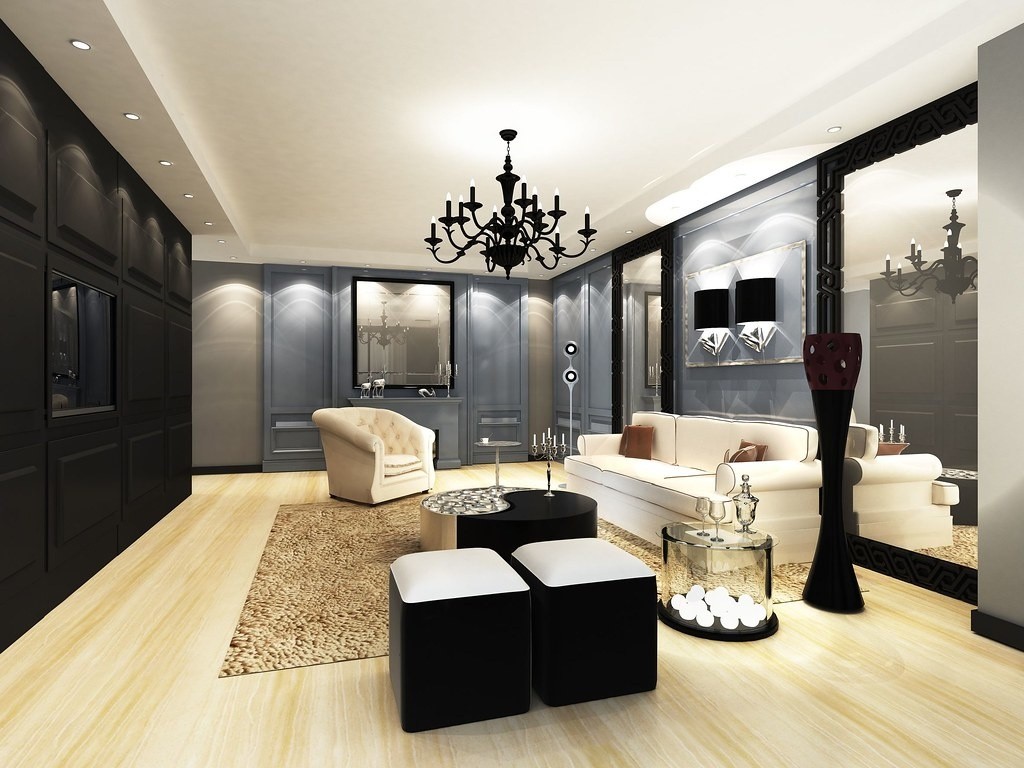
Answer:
[{"left": 0, "top": 16, "right": 194, "bottom": 655}]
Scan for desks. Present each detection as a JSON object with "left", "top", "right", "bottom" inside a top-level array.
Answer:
[
  {"left": 654, "top": 520, "right": 780, "bottom": 643},
  {"left": 420, "top": 485, "right": 597, "bottom": 565}
]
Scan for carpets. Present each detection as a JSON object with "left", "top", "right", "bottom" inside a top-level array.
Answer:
[{"left": 217, "top": 493, "right": 870, "bottom": 681}]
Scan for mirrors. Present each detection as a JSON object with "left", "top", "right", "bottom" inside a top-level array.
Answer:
[
  {"left": 817, "top": 79, "right": 979, "bottom": 607},
  {"left": 644, "top": 291, "right": 661, "bottom": 389},
  {"left": 611, "top": 223, "right": 674, "bottom": 435},
  {"left": 352, "top": 274, "right": 456, "bottom": 392}
]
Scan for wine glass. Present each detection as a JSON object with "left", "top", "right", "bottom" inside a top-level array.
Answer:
[
  {"left": 696, "top": 497, "right": 710, "bottom": 536},
  {"left": 709, "top": 501, "right": 725, "bottom": 542}
]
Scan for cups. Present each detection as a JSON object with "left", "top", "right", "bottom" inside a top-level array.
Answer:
[
  {"left": 361, "top": 383, "right": 371, "bottom": 398},
  {"left": 373, "top": 379, "right": 385, "bottom": 398},
  {"left": 480, "top": 438, "right": 489, "bottom": 443}
]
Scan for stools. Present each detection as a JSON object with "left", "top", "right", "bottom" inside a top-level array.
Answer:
[
  {"left": 510, "top": 538, "right": 658, "bottom": 708},
  {"left": 389, "top": 547, "right": 532, "bottom": 735}
]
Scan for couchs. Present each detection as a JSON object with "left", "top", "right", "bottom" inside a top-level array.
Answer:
[
  {"left": 849, "top": 409, "right": 959, "bottom": 551},
  {"left": 564, "top": 411, "right": 824, "bottom": 574},
  {"left": 311, "top": 408, "right": 435, "bottom": 504}
]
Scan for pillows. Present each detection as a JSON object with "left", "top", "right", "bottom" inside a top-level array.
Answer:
[
  {"left": 618, "top": 424, "right": 655, "bottom": 461},
  {"left": 729, "top": 438, "right": 768, "bottom": 463}
]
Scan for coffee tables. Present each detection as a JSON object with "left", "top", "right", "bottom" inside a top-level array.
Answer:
[{"left": 474, "top": 440, "right": 522, "bottom": 485}]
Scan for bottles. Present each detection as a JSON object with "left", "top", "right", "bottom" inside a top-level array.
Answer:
[{"left": 732, "top": 474, "right": 759, "bottom": 534}]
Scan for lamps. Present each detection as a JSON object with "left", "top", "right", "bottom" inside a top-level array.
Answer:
[
  {"left": 424, "top": 130, "right": 597, "bottom": 281},
  {"left": 879, "top": 188, "right": 978, "bottom": 304},
  {"left": 734, "top": 277, "right": 776, "bottom": 327},
  {"left": 695, "top": 288, "right": 730, "bottom": 331},
  {"left": 358, "top": 301, "right": 410, "bottom": 351}
]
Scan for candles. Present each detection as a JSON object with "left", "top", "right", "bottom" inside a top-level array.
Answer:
[
  {"left": 879, "top": 418, "right": 905, "bottom": 443},
  {"left": 438, "top": 361, "right": 458, "bottom": 376},
  {"left": 533, "top": 426, "right": 566, "bottom": 448},
  {"left": 649, "top": 363, "right": 662, "bottom": 376}
]
